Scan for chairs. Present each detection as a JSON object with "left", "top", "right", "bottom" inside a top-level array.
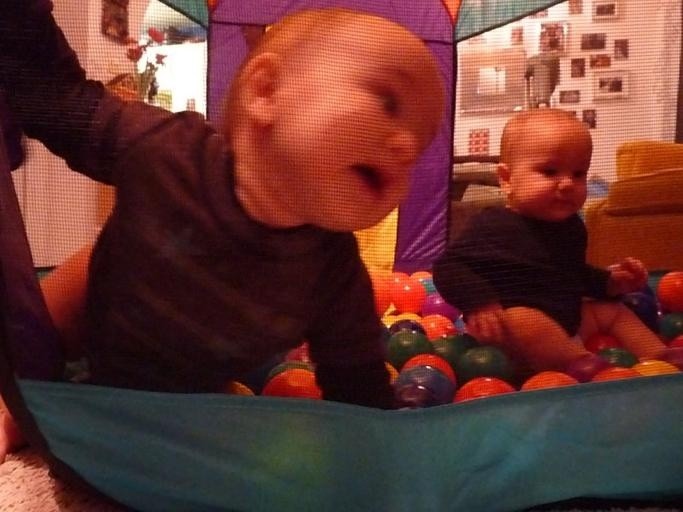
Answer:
[{"left": 582, "top": 140, "right": 683, "bottom": 272}]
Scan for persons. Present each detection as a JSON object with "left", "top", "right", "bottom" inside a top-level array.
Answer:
[
  {"left": 432, "top": 108, "right": 669, "bottom": 385},
  {"left": 0, "top": 0, "right": 443, "bottom": 470}
]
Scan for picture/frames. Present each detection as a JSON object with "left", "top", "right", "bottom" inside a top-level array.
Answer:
[
  {"left": 535, "top": 21, "right": 569, "bottom": 56},
  {"left": 590, "top": 67, "right": 633, "bottom": 102},
  {"left": 590, "top": 0, "right": 622, "bottom": 23}
]
